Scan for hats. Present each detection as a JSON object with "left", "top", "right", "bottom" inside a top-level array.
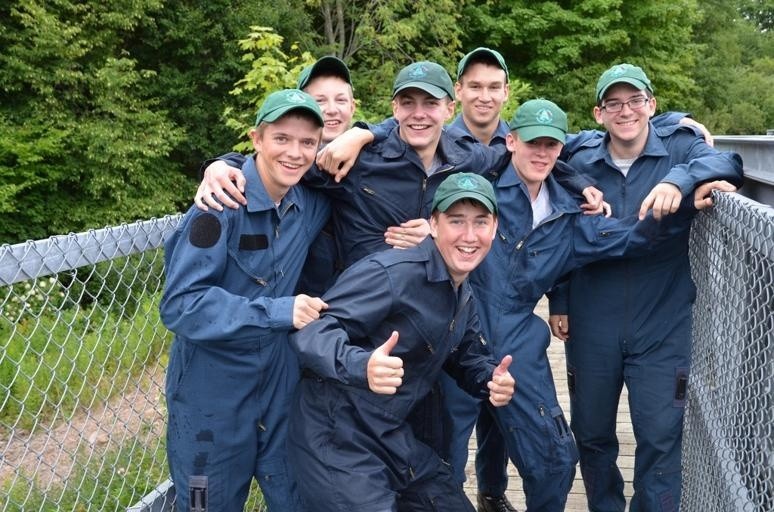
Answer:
[
  {"left": 296, "top": 53, "right": 354, "bottom": 91},
  {"left": 509, "top": 102, "right": 569, "bottom": 147},
  {"left": 430, "top": 171, "right": 499, "bottom": 216},
  {"left": 595, "top": 60, "right": 654, "bottom": 100},
  {"left": 457, "top": 50, "right": 509, "bottom": 87},
  {"left": 255, "top": 87, "right": 326, "bottom": 128},
  {"left": 388, "top": 59, "right": 455, "bottom": 102}
]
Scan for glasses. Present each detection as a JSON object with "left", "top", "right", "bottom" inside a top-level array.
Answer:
[{"left": 597, "top": 98, "right": 653, "bottom": 115}]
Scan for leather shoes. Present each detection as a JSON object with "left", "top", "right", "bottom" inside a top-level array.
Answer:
[{"left": 476, "top": 491, "right": 515, "bottom": 512}]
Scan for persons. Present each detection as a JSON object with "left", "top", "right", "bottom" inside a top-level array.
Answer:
[
  {"left": 293, "top": 55, "right": 373, "bottom": 300},
  {"left": 541, "top": 62, "right": 744, "bottom": 512},
  {"left": 159, "top": 89, "right": 366, "bottom": 512},
  {"left": 194, "top": 60, "right": 612, "bottom": 296},
  {"left": 464, "top": 98, "right": 737, "bottom": 511},
  {"left": 286, "top": 172, "right": 515, "bottom": 512},
  {"left": 315, "top": 48, "right": 714, "bottom": 508}
]
